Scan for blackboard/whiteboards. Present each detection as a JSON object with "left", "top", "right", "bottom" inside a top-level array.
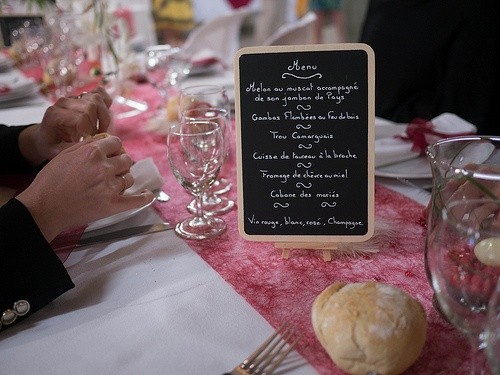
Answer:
[{"left": 233, "top": 43, "right": 376, "bottom": 243}]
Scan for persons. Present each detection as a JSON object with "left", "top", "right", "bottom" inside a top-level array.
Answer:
[{"left": 0, "top": 85, "right": 154, "bottom": 335}]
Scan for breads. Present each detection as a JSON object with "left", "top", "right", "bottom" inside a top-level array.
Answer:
[{"left": 311, "top": 281, "right": 428, "bottom": 374}]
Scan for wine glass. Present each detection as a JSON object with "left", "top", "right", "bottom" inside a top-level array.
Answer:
[
  {"left": 181, "top": 109, "right": 234, "bottom": 215},
  {"left": 166, "top": 121, "right": 227, "bottom": 239},
  {"left": 422, "top": 136, "right": 500, "bottom": 375},
  {"left": 11, "top": 11, "right": 91, "bottom": 101},
  {"left": 177, "top": 83, "right": 233, "bottom": 195},
  {"left": 147, "top": 47, "right": 194, "bottom": 125}
]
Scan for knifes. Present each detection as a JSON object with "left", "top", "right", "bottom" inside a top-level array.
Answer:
[{"left": 51, "top": 222, "right": 176, "bottom": 251}]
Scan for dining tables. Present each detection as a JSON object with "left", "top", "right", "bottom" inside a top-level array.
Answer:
[{"left": 0, "top": 47, "right": 500, "bottom": 375}]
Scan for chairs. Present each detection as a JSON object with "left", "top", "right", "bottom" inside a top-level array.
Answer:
[{"left": 182, "top": 8, "right": 317, "bottom": 69}]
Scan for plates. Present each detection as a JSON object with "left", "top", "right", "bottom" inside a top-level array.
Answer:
[
  {"left": 374, "top": 123, "right": 495, "bottom": 178},
  {"left": 84, "top": 198, "right": 158, "bottom": 232}
]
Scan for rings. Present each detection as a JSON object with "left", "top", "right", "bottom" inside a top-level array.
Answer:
[
  {"left": 117, "top": 175, "right": 126, "bottom": 191},
  {"left": 79, "top": 91, "right": 89, "bottom": 99}
]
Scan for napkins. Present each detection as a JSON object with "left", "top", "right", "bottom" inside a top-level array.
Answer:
[
  {"left": 122, "top": 156, "right": 163, "bottom": 195},
  {"left": 374, "top": 113, "right": 477, "bottom": 169},
  {"left": 0, "top": 67, "right": 34, "bottom": 92}
]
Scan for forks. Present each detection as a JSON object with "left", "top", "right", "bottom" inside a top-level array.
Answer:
[{"left": 222, "top": 321, "right": 304, "bottom": 375}]
